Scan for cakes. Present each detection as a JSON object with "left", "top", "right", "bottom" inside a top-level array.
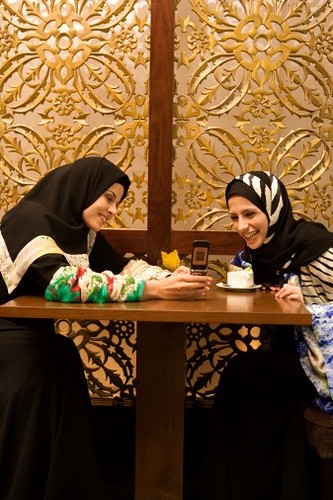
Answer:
[{"left": 227, "top": 268, "right": 254, "bottom": 288}]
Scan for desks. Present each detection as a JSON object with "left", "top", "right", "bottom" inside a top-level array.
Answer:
[{"left": 0, "top": 273, "right": 312, "bottom": 500}]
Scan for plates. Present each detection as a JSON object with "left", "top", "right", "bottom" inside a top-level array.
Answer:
[{"left": 216, "top": 282, "right": 262, "bottom": 292}]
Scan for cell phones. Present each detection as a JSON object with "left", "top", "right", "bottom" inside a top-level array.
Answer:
[{"left": 190, "top": 240, "right": 210, "bottom": 290}]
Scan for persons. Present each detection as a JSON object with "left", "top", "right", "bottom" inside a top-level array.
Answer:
[
  {"left": 196, "top": 170, "right": 332, "bottom": 500},
  {"left": 0, "top": 156, "right": 213, "bottom": 500}
]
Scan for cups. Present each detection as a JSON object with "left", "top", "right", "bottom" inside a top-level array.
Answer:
[{"left": 227, "top": 272, "right": 254, "bottom": 288}]
